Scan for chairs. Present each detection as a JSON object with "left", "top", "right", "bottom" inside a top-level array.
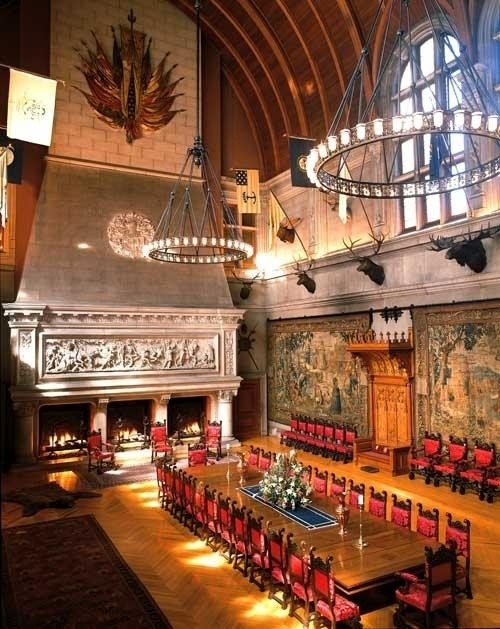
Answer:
[
  {"left": 280, "top": 413, "right": 358, "bottom": 464},
  {"left": 205, "top": 420, "right": 222, "bottom": 460},
  {"left": 150, "top": 419, "right": 173, "bottom": 463},
  {"left": 408, "top": 431, "right": 500, "bottom": 503},
  {"left": 85, "top": 428, "right": 116, "bottom": 474}
]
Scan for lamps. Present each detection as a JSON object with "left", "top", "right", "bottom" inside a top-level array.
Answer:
[
  {"left": 142, "top": 0, "right": 254, "bottom": 265},
  {"left": 306, "top": 0, "right": 500, "bottom": 199}
]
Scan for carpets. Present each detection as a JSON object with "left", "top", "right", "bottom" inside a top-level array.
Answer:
[
  {"left": 73, "top": 448, "right": 240, "bottom": 490},
  {"left": 0, "top": 513, "right": 173, "bottom": 629}
]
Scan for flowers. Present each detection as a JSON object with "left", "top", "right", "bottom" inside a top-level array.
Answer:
[{"left": 251, "top": 450, "right": 315, "bottom": 511}]
